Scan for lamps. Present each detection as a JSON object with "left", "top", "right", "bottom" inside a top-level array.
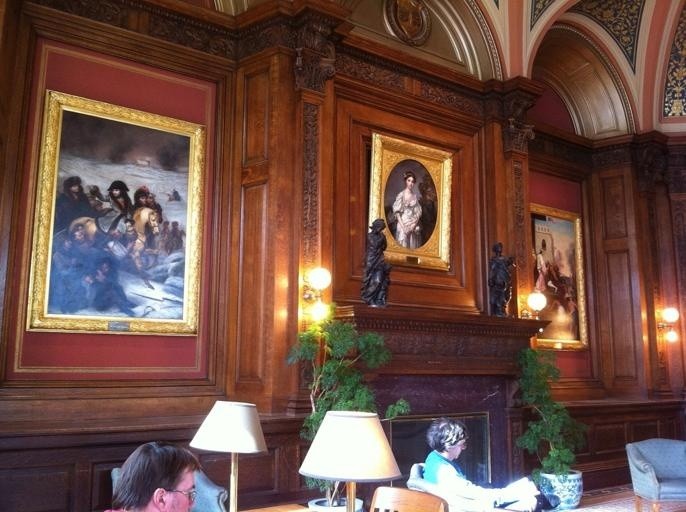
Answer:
[
  {"left": 520, "top": 286, "right": 549, "bottom": 333},
  {"left": 657, "top": 303, "right": 682, "bottom": 347},
  {"left": 186, "top": 397, "right": 267, "bottom": 511},
  {"left": 300, "top": 262, "right": 335, "bottom": 327},
  {"left": 298, "top": 407, "right": 406, "bottom": 512}
]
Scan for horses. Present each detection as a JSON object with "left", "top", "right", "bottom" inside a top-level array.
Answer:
[{"left": 65, "top": 205, "right": 160, "bottom": 291}]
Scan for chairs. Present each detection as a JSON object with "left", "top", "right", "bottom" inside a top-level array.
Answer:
[
  {"left": 368, "top": 485, "right": 448, "bottom": 512},
  {"left": 625, "top": 435, "right": 686, "bottom": 511},
  {"left": 404, "top": 459, "right": 528, "bottom": 512},
  {"left": 107, "top": 465, "right": 229, "bottom": 511}
]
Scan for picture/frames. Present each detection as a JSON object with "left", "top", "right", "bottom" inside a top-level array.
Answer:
[
  {"left": 525, "top": 201, "right": 594, "bottom": 354},
  {"left": 366, "top": 129, "right": 457, "bottom": 274},
  {"left": 19, "top": 86, "right": 211, "bottom": 340}
]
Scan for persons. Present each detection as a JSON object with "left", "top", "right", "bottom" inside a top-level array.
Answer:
[
  {"left": 488, "top": 243, "right": 516, "bottom": 317},
  {"left": 103, "top": 442, "right": 204, "bottom": 512},
  {"left": 392, "top": 171, "right": 422, "bottom": 249},
  {"left": 49, "top": 175, "right": 183, "bottom": 318},
  {"left": 360, "top": 219, "right": 392, "bottom": 306},
  {"left": 423, "top": 416, "right": 541, "bottom": 512}
]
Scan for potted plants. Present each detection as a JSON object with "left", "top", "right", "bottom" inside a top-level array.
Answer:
[
  {"left": 281, "top": 317, "right": 412, "bottom": 512},
  {"left": 508, "top": 343, "right": 593, "bottom": 511}
]
